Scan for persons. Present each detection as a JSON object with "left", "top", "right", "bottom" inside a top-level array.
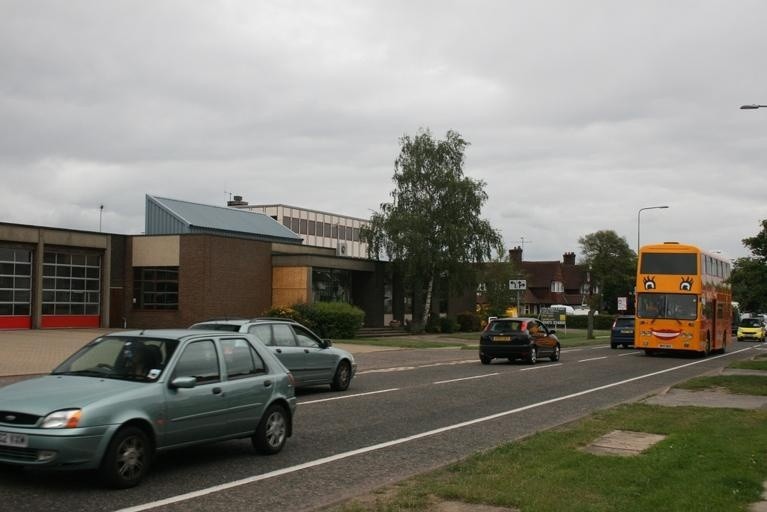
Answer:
[
  {"left": 646, "top": 300, "right": 659, "bottom": 312},
  {"left": 122, "top": 342, "right": 151, "bottom": 377}
]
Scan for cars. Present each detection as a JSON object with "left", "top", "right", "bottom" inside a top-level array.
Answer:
[
  {"left": 611, "top": 314, "right": 635, "bottom": 349},
  {"left": 0, "top": 328, "right": 299, "bottom": 489},
  {"left": 479, "top": 316, "right": 561, "bottom": 365},
  {"left": 738, "top": 317, "right": 767, "bottom": 342},
  {"left": 188, "top": 316, "right": 357, "bottom": 391}
]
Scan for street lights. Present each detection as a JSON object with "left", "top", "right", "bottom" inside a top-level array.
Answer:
[
  {"left": 98, "top": 205, "right": 105, "bottom": 230},
  {"left": 636, "top": 206, "right": 670, "bottom": 253}
]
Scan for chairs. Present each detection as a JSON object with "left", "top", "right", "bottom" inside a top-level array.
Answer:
[{"left": 145, "top": 344, "right": 162, "bottom": 370}]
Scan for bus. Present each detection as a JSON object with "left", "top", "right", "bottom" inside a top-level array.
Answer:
[{"left": 635, "top": 242, "right": 732, "bottom": 357}]
